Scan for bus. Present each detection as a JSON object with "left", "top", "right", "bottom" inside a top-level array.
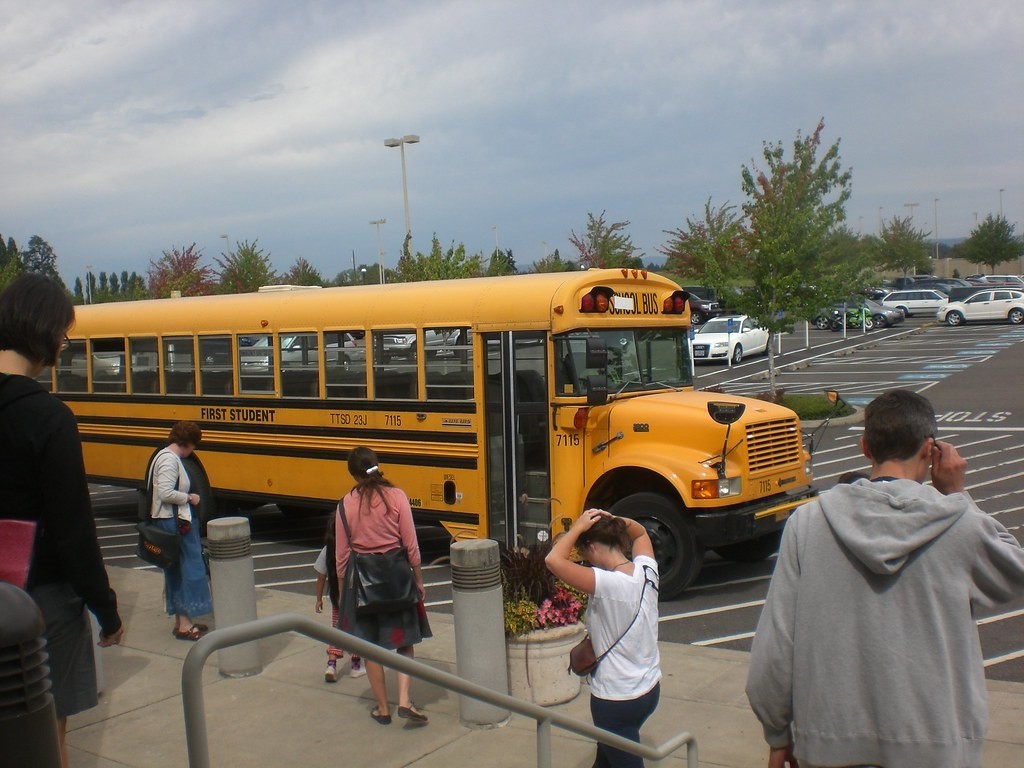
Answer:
[{"left": 31, "top": 264, "right": 818, "bottom": 601}]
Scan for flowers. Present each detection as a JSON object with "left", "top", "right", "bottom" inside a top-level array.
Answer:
[{"left": 501, "top": 530, "right": 593, "bottom": 642}]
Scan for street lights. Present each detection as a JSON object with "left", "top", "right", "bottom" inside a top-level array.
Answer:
[
  {"left": 999, "top": 188, "right": 1005, "bottom": 221},
  {"left": 370, "top": 219, "right": 387, "bottom": 264},
  {"left": 491, "top": 225, "right": 500, "bottom": 276},
  {"left": 903, "top": 202, "right": 919, "bottom": 217},
  {"left": 382, "top": 135, "right": 422, "bottom": 253},
  {"left": 973, "top": 211, "right": 978, "bottom": 228},
  {"left": 220, "top": 234, "right": 231, "bottom": 261},
  {"left": 934, "top": 198, "right": 940, "bottom": 258}
]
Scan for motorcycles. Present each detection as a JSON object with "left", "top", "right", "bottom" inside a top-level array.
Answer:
[{"left": 826, "top": 301, "right": 876, "bottom": 333}]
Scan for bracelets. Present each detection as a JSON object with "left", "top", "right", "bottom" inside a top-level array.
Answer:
[{"left": 187, "top": 494, "right": 193, "bottom": 503}]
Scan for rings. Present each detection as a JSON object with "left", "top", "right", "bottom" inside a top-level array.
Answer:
[{"left": 592, "top": 519, "right": 596, "bottom": 523}]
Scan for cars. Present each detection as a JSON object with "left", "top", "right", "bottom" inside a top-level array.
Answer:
[
  {"left": 808, "top": 270, "right": 1024, "bottom": 328},
  {"left": 189, "top": 336, "right": 253, "bottom": 365},
  {"left": 368, "top": 325, "right": 473, "bottom": 364},
  {"left": 482, "top": 336, "right": 546, "bottom": 346},
  {"left": 239, "top": 331, "right": 367, "bottom": 373},
  {"left": 689, "top": 314, "right": 777, "bottom": 365},
  {"left": 682, "top": 286, "right": 740, "bottom": 327},
  {"left": 53, "top": 348, "right": 136, "bottom": 379}
]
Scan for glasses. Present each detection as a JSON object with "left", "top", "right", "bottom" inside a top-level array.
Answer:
[{"left": 62, "top": 331, "right": 72, "bottom": 352}]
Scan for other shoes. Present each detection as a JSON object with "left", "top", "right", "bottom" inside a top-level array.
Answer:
[
  {"left": 371, "top": 705, "right": 391, "bottom": 725},
  {"left": 350, "top": 664, "right": 366, "bottom": 677},
  {"left": 324, "top": 666, "right": 337, "bottom": 681},
  {"left": 172, "top": 624, "right": 208, "bottom": 641},
  {"left": 398, "top": 700, "right": 428, "bottom": 722}
]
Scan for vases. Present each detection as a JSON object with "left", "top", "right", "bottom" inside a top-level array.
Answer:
[{"left": 505, "top": 620, "right": 586, "bottom": 707}]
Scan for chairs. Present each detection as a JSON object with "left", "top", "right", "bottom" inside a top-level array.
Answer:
[
  {"left": 54, "top": 367, "right": 547, "bottom": 430},
  {"left": 561, "top": 352, "right": 588, "bottom": 393}
]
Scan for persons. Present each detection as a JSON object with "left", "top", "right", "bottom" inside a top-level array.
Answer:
[
  {"left": 745, "top": 388, "right": 1024, "bottom": 768},
  {"left": 147, "top": 421, "right": 213, "bottom": 641},
  {"left": 314, "top": 446, "right": 432, "bottom": 724},
  {"left": 0, "top": 273, "right": 125, "bottom": 768},
  {"left": 545, "top": 508, "right": 662, "bottom": 768}
]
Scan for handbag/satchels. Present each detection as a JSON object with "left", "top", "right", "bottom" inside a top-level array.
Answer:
[
  {"left": 135, "top": 521, "right": 183, "bottom": 572},
  {"left": 567, "top": 639, "right": 597, "bottom": 685},
  {"left": 354, "top": 545, "right": 421, "bottom": 613}
]
